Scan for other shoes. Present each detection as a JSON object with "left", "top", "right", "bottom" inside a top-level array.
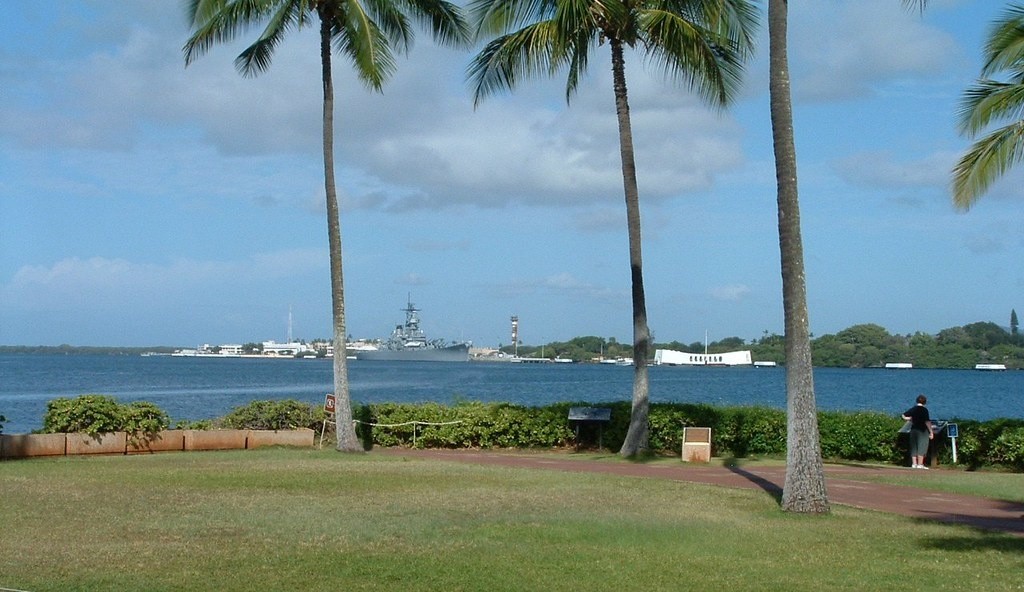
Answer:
[
  {"left": 912, "top": 462, "right": 918, "bottom": 469},
  {"left": 917, "top": 464, "right": 930, "bottom": 470}
]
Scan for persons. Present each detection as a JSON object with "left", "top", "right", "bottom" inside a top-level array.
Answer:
[{"left": 902, "top": 394, "right": 934, "bottom": 470}]
